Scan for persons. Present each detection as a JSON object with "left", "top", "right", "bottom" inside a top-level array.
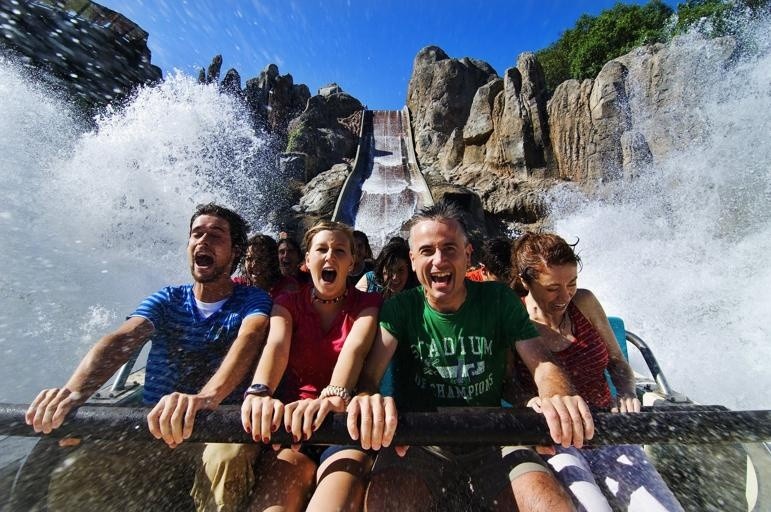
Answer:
[
  {"left": 508, "top": 228, "right": 690, "bottom": 512},
  {"left": 345, "top": 198, "right": 596, "bottom": 511},
  {"left": 239, "top": 218, "right": 383, "bottom": 512},
  {"left": 20, "top": 200, "right": 272, "bottom": 512},
  {"left": 229, "top": 227, "right": 530, "bottom": 463}
]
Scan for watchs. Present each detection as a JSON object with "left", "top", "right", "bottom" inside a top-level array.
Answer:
[{"left": 242, "top": 382, "right": 273, "bottom": 400}]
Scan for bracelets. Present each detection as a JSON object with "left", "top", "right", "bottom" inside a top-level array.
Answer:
[{"left": 317, "top": 386, "right": 351, "bottom": 411}]
